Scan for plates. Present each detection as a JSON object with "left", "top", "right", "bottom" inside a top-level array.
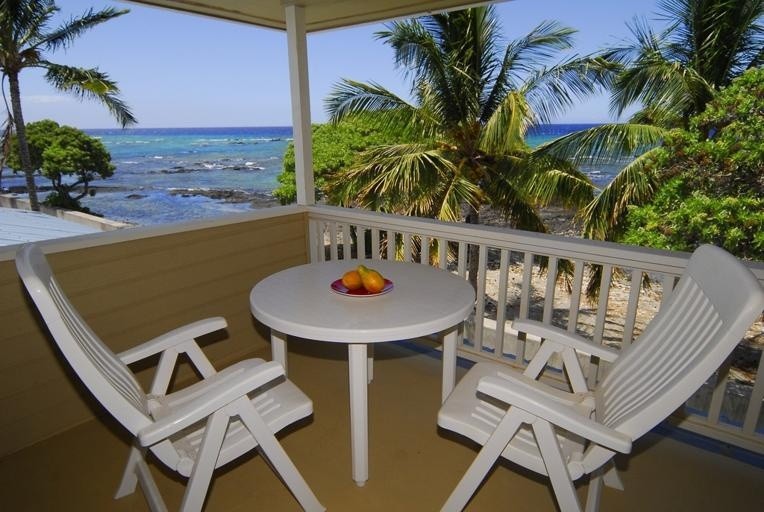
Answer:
[{"left": 330, "top": 278, "right": 394, "bottom": 298}]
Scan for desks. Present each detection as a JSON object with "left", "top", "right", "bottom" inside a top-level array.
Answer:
[{"left": 250, "top": 260, "right": 476, "bottom": 487}]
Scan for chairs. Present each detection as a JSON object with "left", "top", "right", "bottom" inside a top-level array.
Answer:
[
  {"left": 14, "top": 244, "right": 326, "bottom": 512},
  {"left": 437, "top": 244, "right": 764, "bottom": 512}
]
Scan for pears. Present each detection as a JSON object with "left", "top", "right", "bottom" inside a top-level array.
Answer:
[{"left": 342, "top": 265, "right": 385, "bottom": 293}]
